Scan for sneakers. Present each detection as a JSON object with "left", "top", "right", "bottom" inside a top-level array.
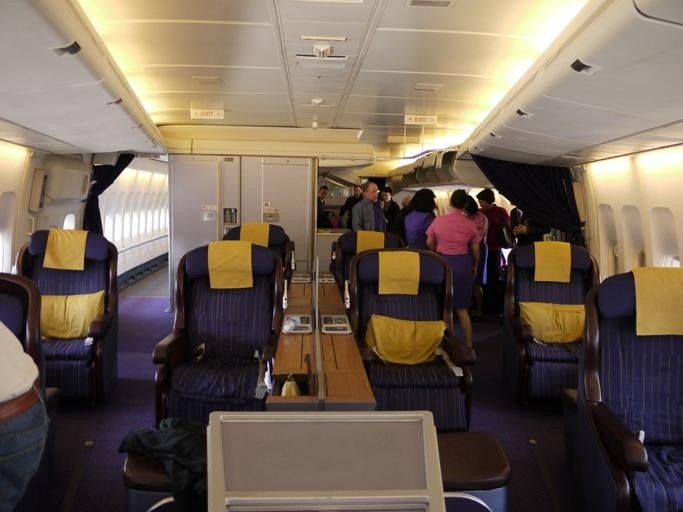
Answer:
[{"left": 462, "top": 348, "right": 477, "bottom": 366}]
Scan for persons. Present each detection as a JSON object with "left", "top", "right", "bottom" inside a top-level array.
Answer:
[
  {"left": 319, "top": 186, "right": 334, "bottom": 229},
  {"left": 508, "top": 207, "right": 545, "bottom": 246},
  {"left": 426, "top": 189, "right": 508, "bottom": 360},
  {"left": 340, "top": 182, "right": 400, "bottom": 231},
  {"left": 1, "top": 319, "right": 51, "bottom": 512},
  {"left": 404, "top": 189, "right": 436, "bottom": 249}
]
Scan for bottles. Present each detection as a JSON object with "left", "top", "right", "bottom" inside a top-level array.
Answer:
[{"left": 282, "top": 315, "right": 302, "bottom": 333}]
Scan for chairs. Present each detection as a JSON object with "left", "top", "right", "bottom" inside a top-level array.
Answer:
[
  {"left": 586, "top": 266, "right": 682, "bottom": 512},
  {"left": 13, "top": 229, "right": 117, "bottom": 408},
  {"left": 2, "top": 274, "right": 41, "bottom": 395},
  {"left": 122, "top": 223, "right": 512, "bottom": 511},
  {"left": 508, "top": 242, "right": 600, "bottom": 403}
]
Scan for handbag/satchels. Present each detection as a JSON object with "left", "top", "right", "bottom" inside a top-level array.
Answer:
[{"left": 495, "top": 219, "right": 512, "bottom": 248}]
